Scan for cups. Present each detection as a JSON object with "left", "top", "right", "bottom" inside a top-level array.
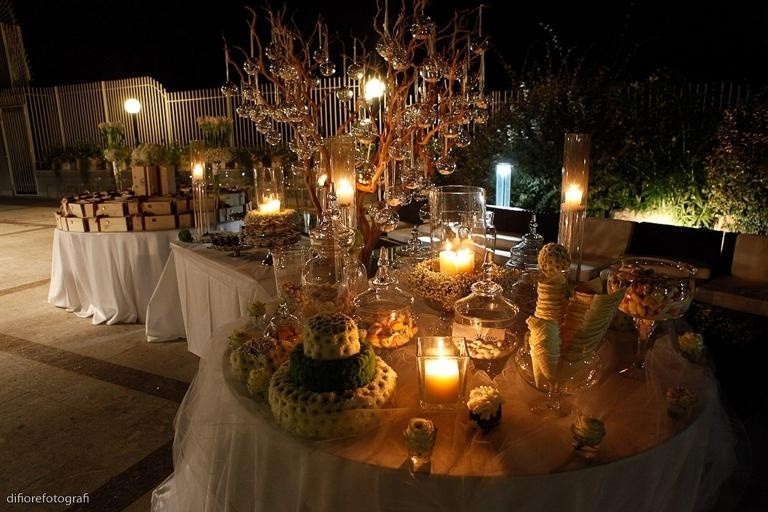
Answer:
[
  {"left": 193, "top": 161, "right": 312, "bottom": 241},
  {"left": 413, "top": 334, "right": 470, "bottom": 409},
  {"left": 265, "top": 183, "right": 629, "bottom": 350}
]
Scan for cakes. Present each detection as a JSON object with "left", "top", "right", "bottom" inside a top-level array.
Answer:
[
  {"left": 466, "top": 385, "right": 502, "bottom": 425},
  {"left": 570, "top": 415, "right": 605, "bottom": 446},
  {"left": 242, "top": 208, "right": 300, "bottom": 248},
  {"left": 229, "top": 312, "right": 397, "bottom": 441},
  {"left": 666, "top": 384, "right": 695, "bottom": 418},
  {"left": 679, "top": 331, "right": 703, "bottom": 363},
  {"left": 403, "top": 418, "right": 437, "bottom": 454}
]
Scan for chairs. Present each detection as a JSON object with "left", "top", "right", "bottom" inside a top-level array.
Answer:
[{"left": 382, "top": 204, "right": 768, "bottom": 316}]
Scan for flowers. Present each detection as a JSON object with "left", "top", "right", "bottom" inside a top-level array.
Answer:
[{"left": 105, "top": 146, "right": 126, "bottom": 161}]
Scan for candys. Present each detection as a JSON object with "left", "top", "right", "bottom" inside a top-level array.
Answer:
[
  {"left": 354, "top": 315, "right": 419, "bottom": 348},
  {"left": 608, "top": 265, "right": 694, "bottom": 321}
]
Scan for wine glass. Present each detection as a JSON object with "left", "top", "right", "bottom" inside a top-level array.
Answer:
[
  {"left": 603, "top": 255, "right": 699, "bottom": 381},
  {"left": 513, "top": 327, "right": 604, "bottom": 421}
]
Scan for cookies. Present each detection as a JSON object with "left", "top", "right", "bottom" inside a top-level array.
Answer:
[{"left": 538, "top": 243, "right": 571, "bottom": 278}]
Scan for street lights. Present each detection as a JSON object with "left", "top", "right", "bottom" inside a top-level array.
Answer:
[{"left": 123, "top": 99, "right": 142, "bottom": 147}]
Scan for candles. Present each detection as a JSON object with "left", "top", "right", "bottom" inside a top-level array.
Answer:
[
  {"left": 457, "top": 248, "right": 473, "bottom": 274},
  {"left": 336, "top": 179, "right": 353, "bottom": 204},
  {"left": 258, "top": 197, "right": 280, "bottom": 215},
  {"left": 565, "top": 182, "right": 583, "bottom": 208},
  {"left": 439, "top": 241, "right": 457, "bottom": 273},
  {"left": 424, "top": 348, "right": 459, "bottom": 401}
]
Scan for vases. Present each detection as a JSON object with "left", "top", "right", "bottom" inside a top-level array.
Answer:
[{"left": 113, "top": 160, "right": 122, "bottom": 193}]
